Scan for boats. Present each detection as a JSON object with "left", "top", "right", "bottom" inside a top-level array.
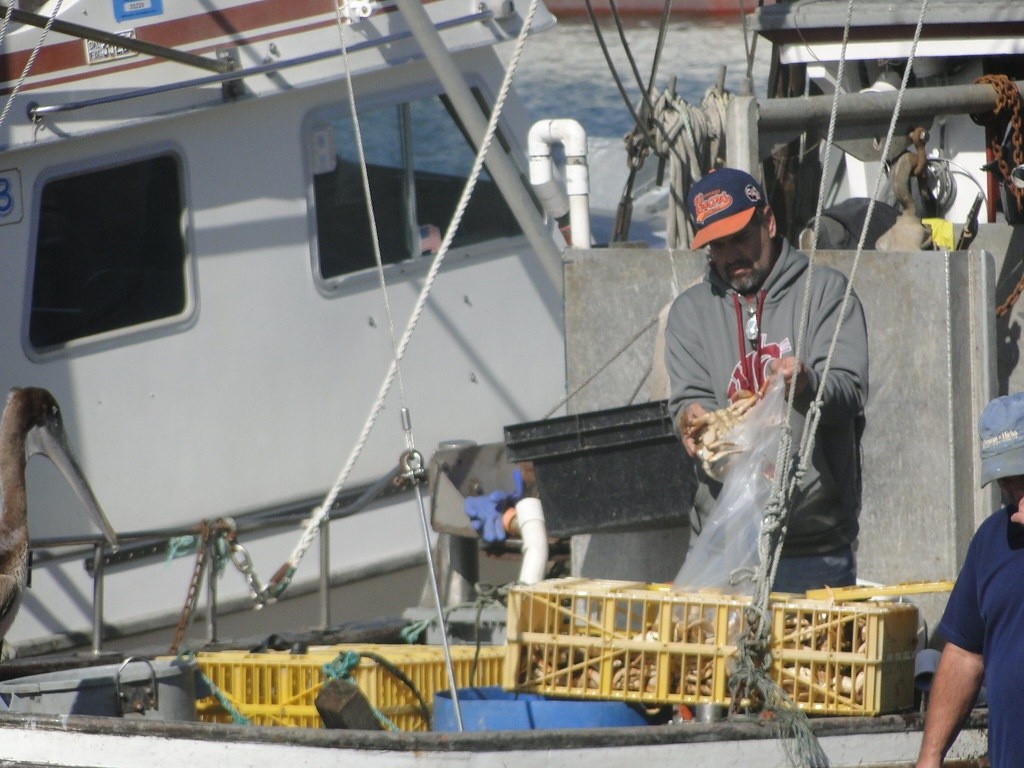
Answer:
[{"left": 1, "top": 0, "right": 597, "bottom": 661}]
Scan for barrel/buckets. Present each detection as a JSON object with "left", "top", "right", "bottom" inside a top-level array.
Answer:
[
  {"left": 433, "top": 686, "right": 650, "bottom": 731},
  {"left": 0, "top": 655, "right": 199, "bottom": 720}
]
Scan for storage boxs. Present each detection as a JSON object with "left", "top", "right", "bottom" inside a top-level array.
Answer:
[
  {"left": 504, "top": 399, "right": 698, "bottom": 537},
  {"left": 0, "top": 576, "right": 957, "bottom": 733}
]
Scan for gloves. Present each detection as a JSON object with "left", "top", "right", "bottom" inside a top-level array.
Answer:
[{"left": 461, "top": 469, "right": 523, "bottom": 542}]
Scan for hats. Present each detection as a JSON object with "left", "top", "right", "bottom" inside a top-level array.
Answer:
[
  {"left": 687, "top": 170, "right": 767, "bottom": 251},
  {"left": 978, "top": 393, "right": 1024, "bottom": 489}
]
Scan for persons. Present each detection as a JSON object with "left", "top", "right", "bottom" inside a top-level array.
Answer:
[
  {"left": 915, "top": 391, "right": 1024, "bottom": 768},
  {"left": 666, "top": 169, "right": 868, "bottom": 592}
]
